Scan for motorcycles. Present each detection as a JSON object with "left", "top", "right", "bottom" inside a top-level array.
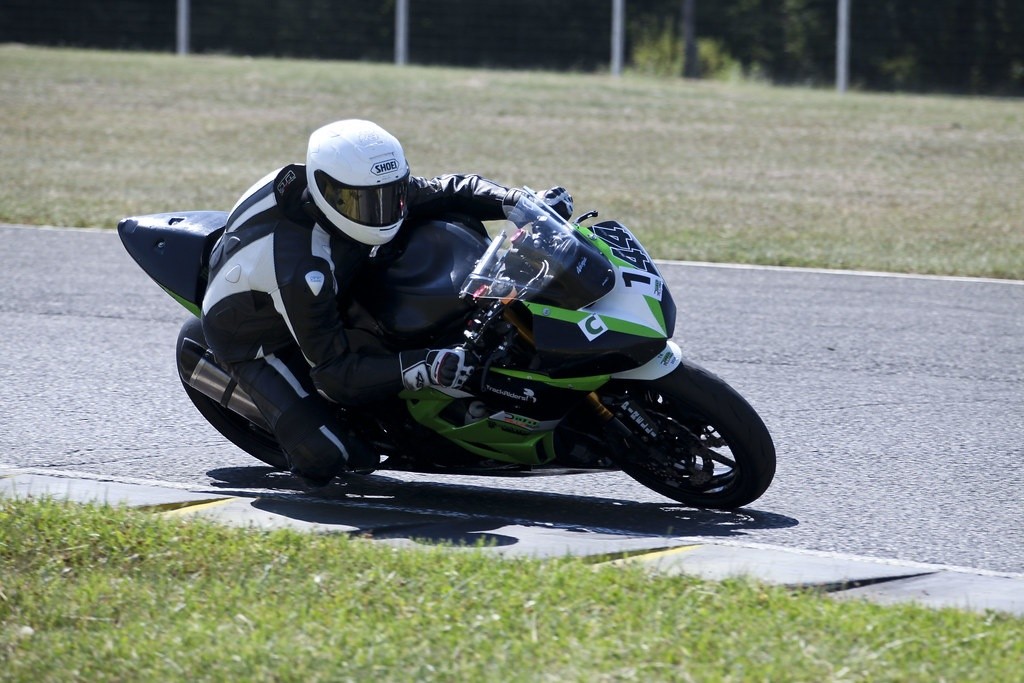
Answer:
[{"left": 113, "top": 183, "right": 781, "bottom": 512}]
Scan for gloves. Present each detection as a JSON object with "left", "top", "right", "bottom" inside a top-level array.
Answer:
[
  {"left": 399, "top": 346, "right": 479, "bottom": 391},
  {"left": 502, "top": 186, "right": 575, "bottom": 224}
]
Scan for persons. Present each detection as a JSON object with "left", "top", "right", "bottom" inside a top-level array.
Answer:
[{"left": 199, "top": 117, "right": 576, "bottom": 486}]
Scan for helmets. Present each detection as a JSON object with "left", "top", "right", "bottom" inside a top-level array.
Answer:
[{"left": 306, "top": 119, "right": 409, "bottom": 245}]
